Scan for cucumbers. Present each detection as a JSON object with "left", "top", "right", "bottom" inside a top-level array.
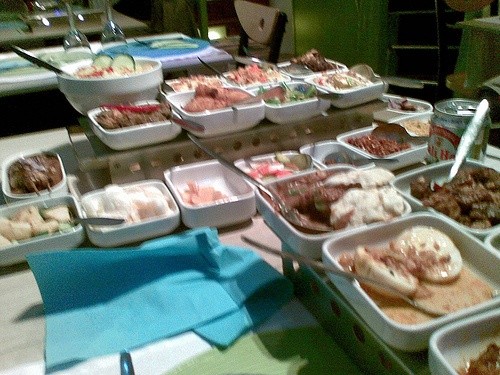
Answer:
[
  {"left": 110, "top": 54, "right": 135, "bottom": 71},
  {"left": 93, "top": 54, "right": 114, "bottom": 69}
]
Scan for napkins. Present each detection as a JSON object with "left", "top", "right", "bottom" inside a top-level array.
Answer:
[{"left": 24, "top": 226, "right": 295, "bottom": 375}]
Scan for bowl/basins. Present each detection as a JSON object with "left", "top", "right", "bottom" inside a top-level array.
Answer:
[
  {"left": 0, "top": 194, "right": 84, "bottom": 269},
  {"left": 79, "top": 180, "right": 181, "bottom": 248},
  {"left": 85, "top": 55, "right": 389, "bottom": 152},
  {"left": 233, "top": 93, "right": 500, "bottom": 375},
  {"left": 164, "top": 159, "right": 256, "bottom": 231},
  {"left": 56, "top": 56, "right": 163, "bottom": 116},
  {"left": 0, "top": 148, "right": 69, "bottom": 203}
]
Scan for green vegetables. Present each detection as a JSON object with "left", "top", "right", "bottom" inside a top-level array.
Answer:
[{"left": 258, "top": 81, "right": 317, "bottom": 105}]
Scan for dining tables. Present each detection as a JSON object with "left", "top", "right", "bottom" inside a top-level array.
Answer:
[{"left": 0, "top": 90, "right": 500, "bottom": 375}]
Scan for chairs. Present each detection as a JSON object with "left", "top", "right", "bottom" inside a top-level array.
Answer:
[{"left": 234, "top": 0, "right": 288, "bottom": 63}]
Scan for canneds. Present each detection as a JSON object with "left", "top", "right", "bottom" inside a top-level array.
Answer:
[{"left": 424, "top": 98, "right": 491, "bottom": 165}]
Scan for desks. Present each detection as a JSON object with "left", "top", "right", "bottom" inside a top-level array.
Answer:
[{"left": 0, "top": 9, "right": 233, "bottom": 96}]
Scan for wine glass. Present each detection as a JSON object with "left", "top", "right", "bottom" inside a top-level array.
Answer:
[
  {"left": 101, "top": 0, "right": 128, "bottom": 53},
  {"left": 61, "top": 0, "right": 93, "bottom": 59}
]
